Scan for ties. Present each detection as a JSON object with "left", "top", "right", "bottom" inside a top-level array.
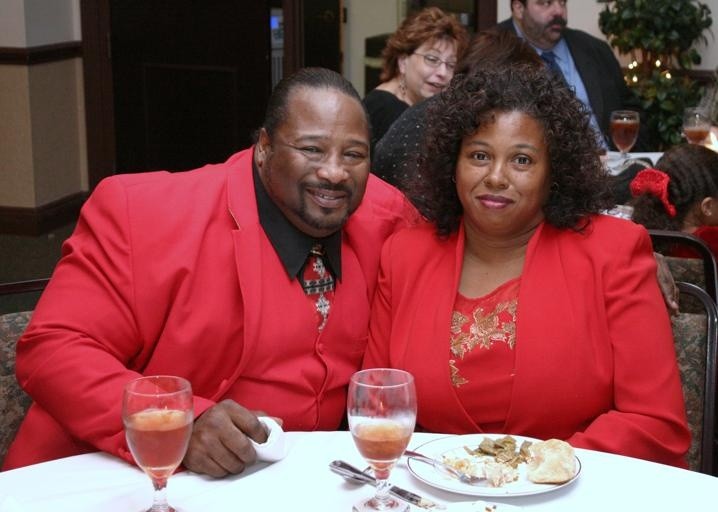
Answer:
[
  {"left": 302, "top": 243, "right": 336, "bottom": 332},
  {"left": 543, "top": 54, "right": 570, "bottom": 106}
]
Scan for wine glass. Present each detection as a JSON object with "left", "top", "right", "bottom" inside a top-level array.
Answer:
[
  {"left": 347, "top": 368, "right": 418, "bottom": 512},
  {"left": 122, "top": 375, "right": 194, "bottom": 512},
  {"left": 609, "top": 111, "right": 640, "bottom": 166},
  {"left": 682, "top": 106, "right": 711, "bottom": 145}
]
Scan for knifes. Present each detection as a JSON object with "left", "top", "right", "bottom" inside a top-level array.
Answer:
[{"left": 329, "top": 459, "right": 447, "bottom": 511}]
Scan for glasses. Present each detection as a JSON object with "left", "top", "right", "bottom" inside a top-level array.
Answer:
[{"left": 411, "top": 51, "right": 456, "bottom": 75}]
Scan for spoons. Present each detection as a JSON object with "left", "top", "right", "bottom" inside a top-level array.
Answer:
[{"left": 342, "top": 466, "right": 372, "bottom": 484}]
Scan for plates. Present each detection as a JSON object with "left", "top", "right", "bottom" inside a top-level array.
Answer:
[{"left": 404, "top": 432, "right": 583, "bottom": 497}]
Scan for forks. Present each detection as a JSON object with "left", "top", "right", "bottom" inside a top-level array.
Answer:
[{"left": 404, "top": 449, "right": 487, "bottom": 483}]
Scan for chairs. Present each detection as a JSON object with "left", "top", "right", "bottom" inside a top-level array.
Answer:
[
  {"left": 644, "top": 229, "right": 714, "bottom": 310},
  {"left": 0, "top": 275, "right": 56, "bottom": 473},
  {"left": 658, "top": 285, "right": 718, "bottom": 474}
]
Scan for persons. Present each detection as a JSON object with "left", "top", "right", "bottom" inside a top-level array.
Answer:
[
  {"left": 361, "top": 5, "right": 470, "bottom": 170},
  {"left": 485, "top": 0, "right": 662, "bottom": 157},
  {"left": 695, "top": 85, "right": 718, "bottom": 152},
  {"left": 625, "top": 141, "right": 716, "bottom": 264},
  {"left": 359, "top": 56, "right": 695, "bottom": 468},
  {"left": 2, "top": 66, "right": 682, "bottom": 472}
]
